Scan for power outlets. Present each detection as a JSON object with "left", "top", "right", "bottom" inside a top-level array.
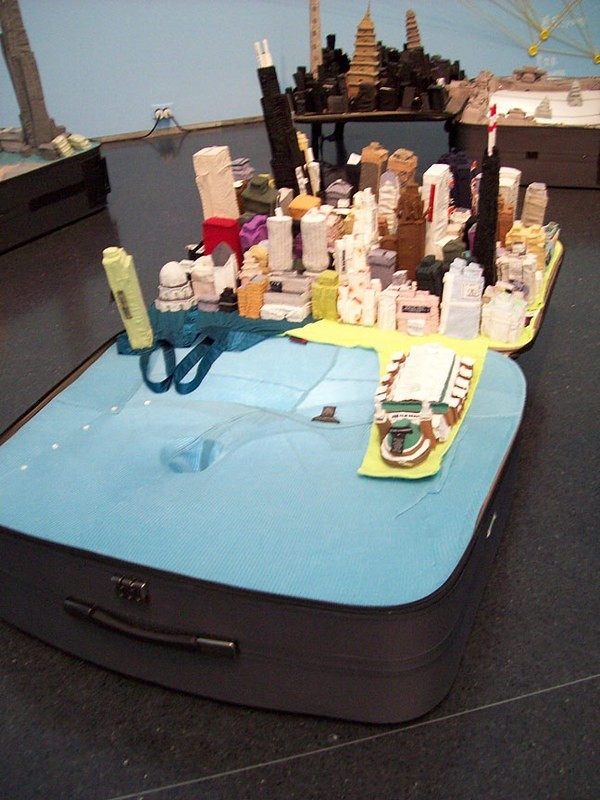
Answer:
[{"left": 153, "top": 103, "right": 175, "bottom": 120}]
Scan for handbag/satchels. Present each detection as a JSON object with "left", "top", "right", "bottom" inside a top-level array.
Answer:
[{"left": 115, "top": 301, "right": 319, "bottom": 395}]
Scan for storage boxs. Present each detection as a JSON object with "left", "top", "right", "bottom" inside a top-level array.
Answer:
[{"left": 451, "top": 71, "right": 600, "bottom": 191}]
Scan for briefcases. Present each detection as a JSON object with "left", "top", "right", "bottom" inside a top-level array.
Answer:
[
  {"left": 0, "top": 138, "right": 112, "bottom": 255},
  {"left": 450, "top": 72, "right": 600, "bottom": 187},
  {"left": 0, "top": 224, "right": 564, "bottom": 721}
]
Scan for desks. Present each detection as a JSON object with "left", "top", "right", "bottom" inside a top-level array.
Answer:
[{"left": 293, "top": 109, "right": 452, "bottom": 167}]
